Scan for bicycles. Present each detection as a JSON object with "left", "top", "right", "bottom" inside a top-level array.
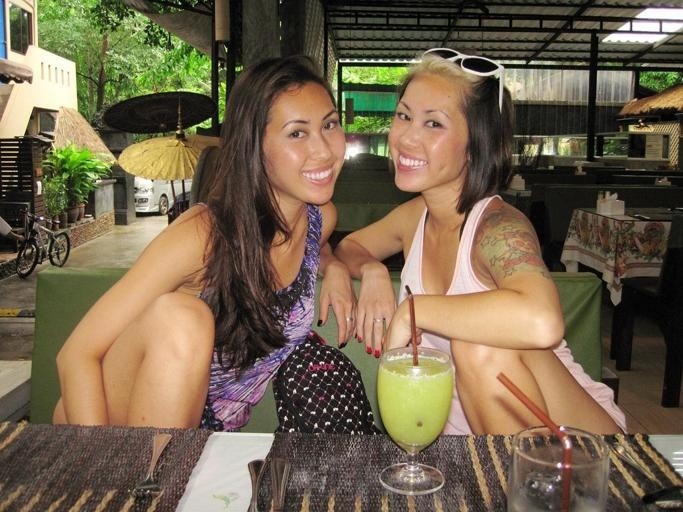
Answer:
[{"left": 15, "top": 207, "right": 70, "bottom": 279}]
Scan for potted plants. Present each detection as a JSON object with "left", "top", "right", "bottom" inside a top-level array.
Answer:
[
  {"left": 58, "top": 175, "right": 68, "bottom": 228},
  {"left": 40, "top": 140, "right": 96, "bottom": 222},
  {"left": 49, "top": 183, "right": 69, "bottom": 232},
  {"left": 40, "top": 177, "right": 59, "bottom": 230},
  {"left": 78, "top": 146, "right": 114, "bottom": 220}
]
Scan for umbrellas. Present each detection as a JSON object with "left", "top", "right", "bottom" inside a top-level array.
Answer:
[
  {"left": 117, "top": 96, "right": 220, "bottom": 210},
  {"left": 101, "top": 91, "right": 218, "bottom": 216}
]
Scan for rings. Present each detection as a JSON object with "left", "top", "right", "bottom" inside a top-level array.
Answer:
[
  {"left": 346, "top": 316, "right": 354, "bottom": 321},
  {"left": 374, "top": 318, "right": 384, "bottom": 322}
]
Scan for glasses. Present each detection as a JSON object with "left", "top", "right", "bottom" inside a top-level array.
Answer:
[{"left": 422, "top": 48, "right": 505, "bottom": 114}]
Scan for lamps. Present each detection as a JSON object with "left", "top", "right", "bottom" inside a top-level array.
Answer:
[
  {"left": 346, "top": 85, "right": 355, "bottom": 124},
  {"left": 215, "top": 0, "right": 231, "bottom": 44}
]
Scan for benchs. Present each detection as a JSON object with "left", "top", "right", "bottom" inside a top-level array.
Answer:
[{"left": 31, "top": 265, "right": 612, "bottom": 442}]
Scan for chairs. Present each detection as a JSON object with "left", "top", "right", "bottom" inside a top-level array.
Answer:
[
  {"left": 616, "top": 215, "right": 683, "bottom": 407},
  {"left": 0, "top": 202, "right": 31, "bottom": 252}
]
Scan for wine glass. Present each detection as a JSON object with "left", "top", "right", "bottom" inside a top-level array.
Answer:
[{"left": 377, "top": 348, "right": 455, "bottom": 496}]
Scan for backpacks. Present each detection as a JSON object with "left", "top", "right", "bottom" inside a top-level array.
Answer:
[{"left": 270, "top": 330, "right": 381, "bottom": 435}]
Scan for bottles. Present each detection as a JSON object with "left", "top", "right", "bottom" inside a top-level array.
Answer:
[{"left": 596, "top": 191, "right": 603, "bottom": 214}]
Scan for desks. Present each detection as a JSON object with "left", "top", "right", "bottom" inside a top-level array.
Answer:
[
  {"left": 559, "top": 205, "right": 683, "bottom": 359},
  {"left": 0, "top": 420, "right": 683, "bottom": 512},
  {"left": 331, "top": 158, "right": 421, "bottom": 234},
  {"left": 500, "top": 165, "right": 683, "bottom": 244}
]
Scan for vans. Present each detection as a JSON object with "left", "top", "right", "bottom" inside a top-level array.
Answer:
[{"left": 134, "top": 173, "right": 192, "bottom": 216}]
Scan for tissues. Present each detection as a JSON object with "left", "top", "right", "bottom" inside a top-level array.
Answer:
[
  {"left": 657, "top": 177, "right": 671, "bottom": 185},
  {"left": 596, "top": 191, "right": 625, "bottom": 216},
  {"left": 510, "top": 174, "right": 525, "bottom": 190}
]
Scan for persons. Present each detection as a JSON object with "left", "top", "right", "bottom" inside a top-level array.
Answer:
[
  {"left": 336, "top": 51, "right": 627, "bottom": 436},
  {"left": 51, "top": 56, "right": 357, "bottom": 431},
  {"left": 0, "top": 216, "right": 24, "bottom": 242}
]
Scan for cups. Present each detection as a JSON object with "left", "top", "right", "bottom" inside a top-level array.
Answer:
[{"left": 509, "top": 426, "right": 611, "bottom": 512}]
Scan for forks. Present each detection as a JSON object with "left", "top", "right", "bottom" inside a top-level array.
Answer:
[{"left": 131, "top": 434, "right": 173, "bottom": 497}]
